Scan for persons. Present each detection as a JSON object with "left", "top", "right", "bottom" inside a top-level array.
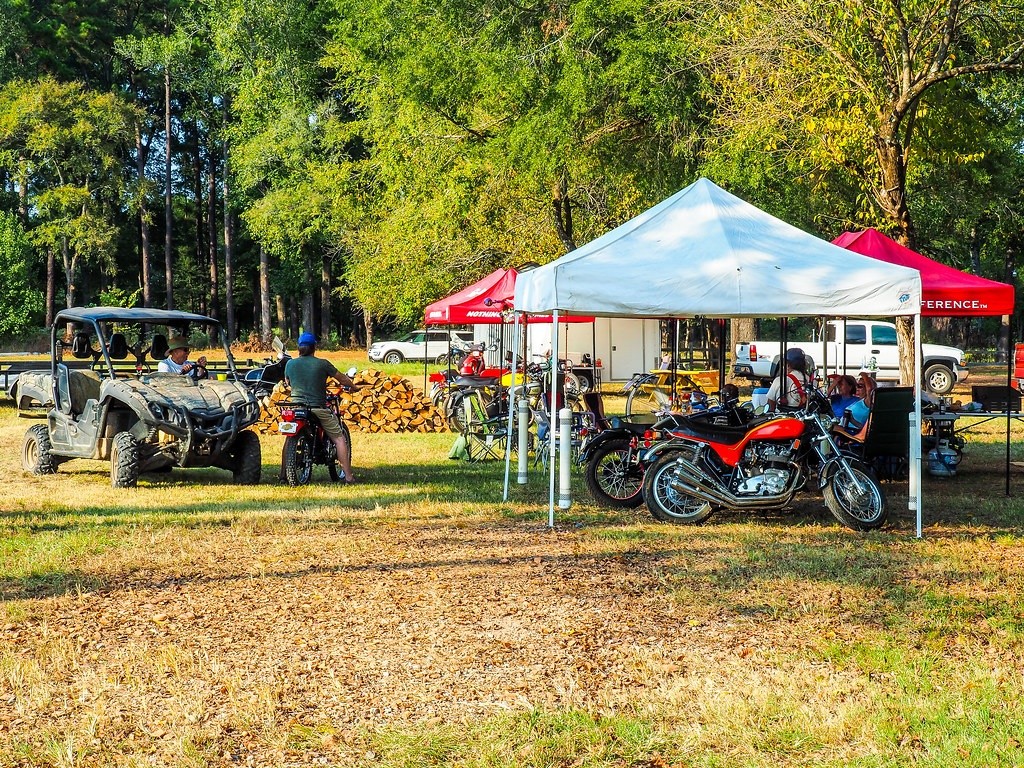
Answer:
[
  {"left": 766, "top": 348, "right": 877, "bottom": 491},
  {"left": 157, "top": 336, "right": 208, "bottom": 379},
  {"left": 284, "top": 333, "right": 360, "bottom": 485}
]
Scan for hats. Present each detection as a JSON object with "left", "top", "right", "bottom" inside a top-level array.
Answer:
[
  {"left": 164, "top": 337, "right": 197, "bottom": 357},
  {"left": 787, "top": 348, "right": 805, "bottom": 361},
  {"left": 298, "top": 331, "right": 317, "bottom": 346}
]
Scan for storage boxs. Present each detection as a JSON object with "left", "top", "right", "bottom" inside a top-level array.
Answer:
[{"left": 972, "top": 385, "right": 1021, "bottom": 413}]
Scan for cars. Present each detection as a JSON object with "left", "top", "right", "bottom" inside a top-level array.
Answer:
[
  {"left": 368, "top": 330, "right": 474, "bottom": 366},
  {"left": 19, "top": 305, "right": 261, "bottom": 490}
]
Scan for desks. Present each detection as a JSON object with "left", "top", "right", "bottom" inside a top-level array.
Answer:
[
  {"left": 920, "top": 414, "right": 960, "bottom": 478},
  {"left": 567, "top": 367, "right": 605, "bottom": 393},
  {"left": 933, "top": 412, "right": 1024, "bottom": 434},
  {"left": 647, "top": 369, "right": 719, "bottom": 402}
]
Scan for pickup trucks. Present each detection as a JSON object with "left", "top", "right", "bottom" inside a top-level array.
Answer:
[{"left": 730, "top": 320, "right": 970, "bottom": 396}]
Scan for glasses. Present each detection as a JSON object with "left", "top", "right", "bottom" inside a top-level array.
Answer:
[
  {"left": 176, "top": 348, "right": 191, "bottom": 352},
  {"left": 855, "top": 383, "right": 865, "bottom": 388}
]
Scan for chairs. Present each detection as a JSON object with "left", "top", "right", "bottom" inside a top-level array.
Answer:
[
  {"left": 463, "top": 395, "right": 507, "bottom": 465},
  {"left": 528, "top": 404, "right": 584, "bottom": 477},
  {"left": 834, "top": 387, "right": 910, "bottom": 482},
  {"left": 582, "top": 392, "right": 612, "bottom": 432},
  {"left": 470, "top": 395, "right": 518, "bottom": 453},
  {"left": 69, "top": 369, "right": 102, "bottom": 416}
]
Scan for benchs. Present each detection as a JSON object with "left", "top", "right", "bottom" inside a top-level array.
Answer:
[{"left": 640, "top": 383, "right": 683, "bottom": 394}]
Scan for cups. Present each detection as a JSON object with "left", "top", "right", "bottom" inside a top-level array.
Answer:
[{"left": 217, "top": 374, "right": 227, "bottom": 380}]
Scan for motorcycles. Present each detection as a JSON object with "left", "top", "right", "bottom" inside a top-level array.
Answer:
[
  {"left": 434, "top": 344, "right": 465, "bottom": 365},
  {"left": 575, "top": 368, "right": 890, "bottom": 531},
  {"left": 273, "top": 367, "right": 358, "bottom": 488},
  {"left": 430, "top": 338, "right": 581, "bottom": 435}
]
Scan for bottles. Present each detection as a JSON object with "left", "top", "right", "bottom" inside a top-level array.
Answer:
[{"left": 939, "top": 399, "right": 945, "bottom": 415}]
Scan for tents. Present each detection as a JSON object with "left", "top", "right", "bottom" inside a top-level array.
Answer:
[
  {"left": 424, "top": 269, "right": 597, "bottom": 432},
  {"left": 501, "top": 176, "right": 924, "bottom": 539},
  {"left": 781, "top": 228, "right": 1014, "bottom": 496}
]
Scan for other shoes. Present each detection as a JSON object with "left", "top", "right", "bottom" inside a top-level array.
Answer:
[
  {"left": 278, "top": 477, "right": 288, "bottom": 485},
  {"left": 344, "top": 477, "right": 365, "bottom": 485}
]
